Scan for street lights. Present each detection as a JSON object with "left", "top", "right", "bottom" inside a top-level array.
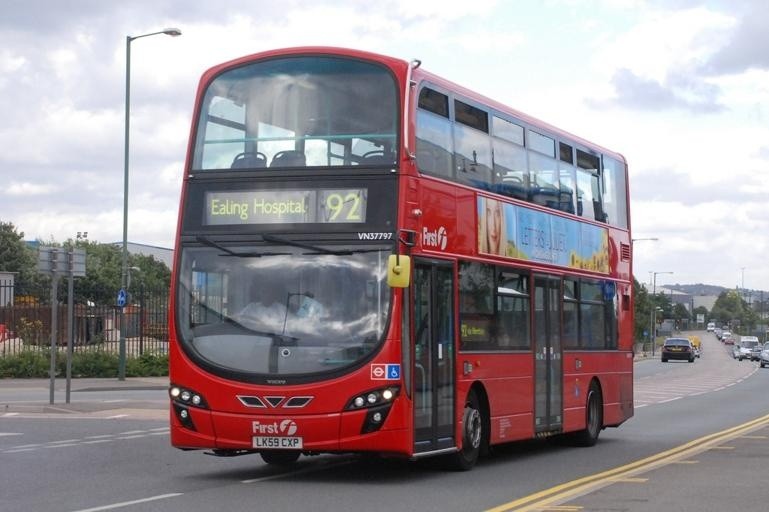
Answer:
[
  {"left": 631, "top": 237, "right": 659, "bottom": 259},
  {"left": 654, "top": 309, "right": 665, "bottom": 351},
  {"left": 118, "top": 28, "right": 182, "bottom": 381},
  {"left": 652, "top": 270, "right": 675, "bottom": 355}
]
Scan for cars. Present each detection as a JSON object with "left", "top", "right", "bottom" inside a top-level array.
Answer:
[
  {"left": 707, "top": 323, "right": 769, "bottom": 369},
  {"left": 661, "top": 337, "right": 694, "bottom": 363}
]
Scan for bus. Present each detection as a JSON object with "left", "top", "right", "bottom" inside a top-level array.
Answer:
[{"left": 168, "top": 45, "right": 635, "bottom": 475}]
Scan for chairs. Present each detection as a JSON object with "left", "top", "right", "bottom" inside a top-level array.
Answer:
[
  {"left": 230, "top": 150, "right": 306, "bottom": 169},
  {"left": 362, "top": 150, "right": 388, "bottom": 168},
  {"left": 496, "top": 176, "right": 607, "bottom": 223}
]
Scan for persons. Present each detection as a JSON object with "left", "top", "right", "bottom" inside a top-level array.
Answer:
[
  {"left": 477, "top": 196, "right": 508, "bottom": 257},
  {"left": 230, "top": 276, "right": 295, "bottom": 325}
]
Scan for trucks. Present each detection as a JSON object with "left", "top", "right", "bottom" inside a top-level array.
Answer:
[{"left": 689, "top": 335, "right": 701, "bottom": 358}]
